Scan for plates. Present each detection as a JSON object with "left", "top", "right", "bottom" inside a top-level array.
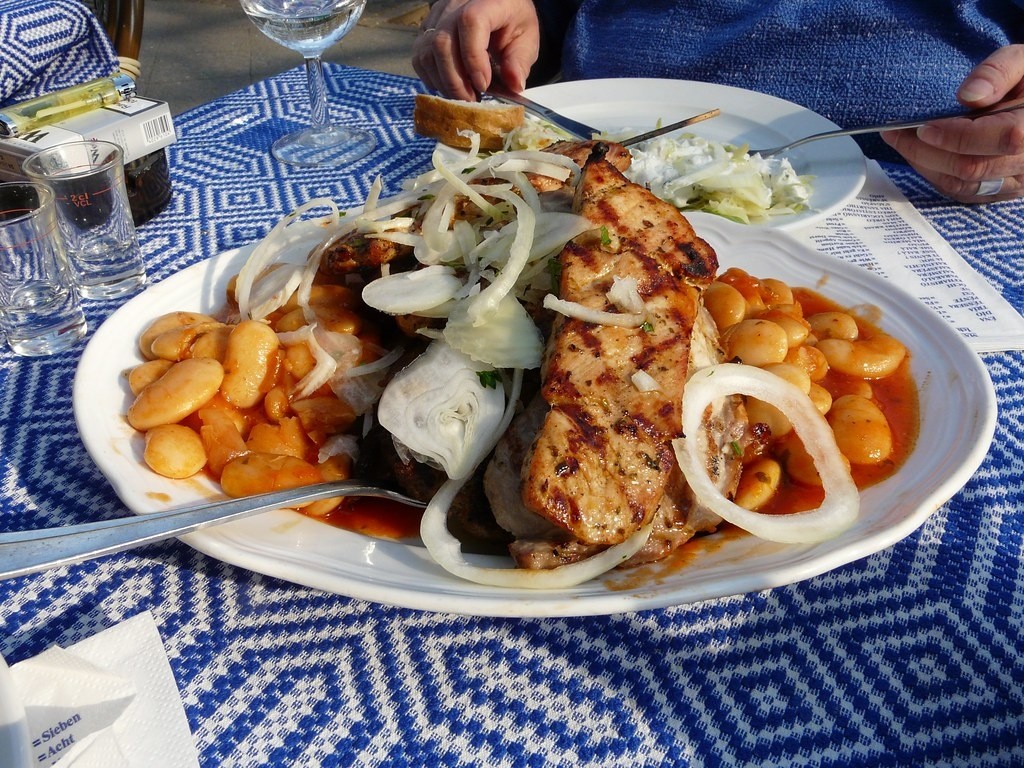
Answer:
[
  {"left": 72, "top": 211, "right": 998, "bottom": 617},
  {"left": 489, "top": 77, "right": 868, "bottom": 231}
]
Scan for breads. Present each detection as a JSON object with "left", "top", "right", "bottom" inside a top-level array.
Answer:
[{"left": 413, "top": 94, "right": 527, "bottom": 150}]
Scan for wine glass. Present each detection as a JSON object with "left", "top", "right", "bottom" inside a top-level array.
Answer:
[{"left": 240, "top": 0, "right": 377, "bottom": 167}]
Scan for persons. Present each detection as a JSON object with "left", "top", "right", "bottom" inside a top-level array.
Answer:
[{"left": 410, "top": 0, "right": 1024, "bottom": 205}]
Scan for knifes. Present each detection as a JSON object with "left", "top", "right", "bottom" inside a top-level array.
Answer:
[{"left": 473, "top": 80, "right": 601, "bottom": 141}]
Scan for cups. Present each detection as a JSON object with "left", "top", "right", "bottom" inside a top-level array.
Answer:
[
  {"left": 22, "top": 140, "right": 147, "bottom": 302},
  {"left": 0, "top": 182, "right": 88, "bottom": 358}
]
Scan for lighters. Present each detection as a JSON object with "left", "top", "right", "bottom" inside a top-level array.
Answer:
[{"left": 0, "top": 74, "right": 137, "bottom": 140}]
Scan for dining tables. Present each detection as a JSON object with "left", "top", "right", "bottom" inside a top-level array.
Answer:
[{"left": 1, "top": 63, "right": 1024, "bottom": 768}]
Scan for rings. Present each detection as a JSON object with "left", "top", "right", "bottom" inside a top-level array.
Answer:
[
  {"left": 424, "top": 28, "right": 435, "bottom": 34},
  {"left": 975, "top": 178, "right": 1004, "bottom": 196}
]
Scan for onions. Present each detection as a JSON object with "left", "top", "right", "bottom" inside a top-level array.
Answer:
[{"left": 229, "top": 128, "right": 862, "bottom": 589}]
